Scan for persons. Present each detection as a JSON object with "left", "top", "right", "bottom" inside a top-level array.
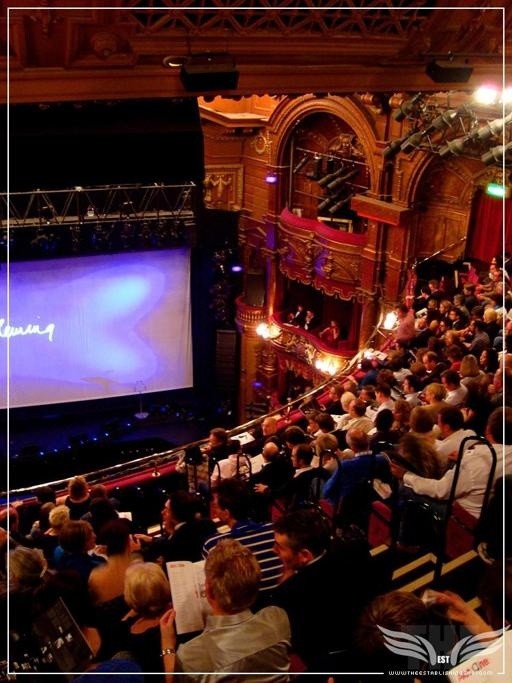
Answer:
[{"left": 0, "top": 251, "right": 512, "bottom": 683}]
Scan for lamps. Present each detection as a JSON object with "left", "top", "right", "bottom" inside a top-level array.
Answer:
[{"left": 291, "top": 92, "right": 512, "bottom": 214}]
